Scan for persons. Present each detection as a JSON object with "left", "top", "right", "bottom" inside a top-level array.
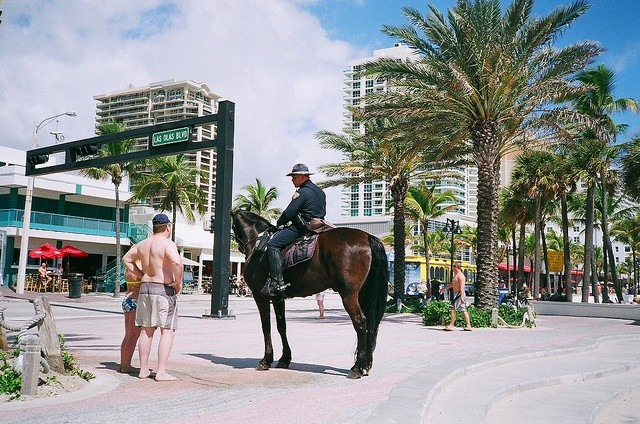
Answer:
[
  {"left": 38, "top": 262, "right": 52, "bottom": 288},
  {"left": 596, "top": 282, "right": 612, "bottom": 298},
  {"left": 120, "top": 244, "right": 144, "bottom": 374},
  {"left": 417, "top": 280, "right": 427, "bottom": 300},
  {"left": 498, "top": 279, "right": 507, "bottom": 288},
  {"left": 52, "top": 263, "right": 64, "bottom": 289},
  {"left": 439, "top": 262, "right": 473, "bottom": 332},
  {"left": 258, "top": 162, "right": 327, "bottom": 298},
  {"left": 316, "top": 291, "right": 325, "bottom": 320},
  {"left": 429, "top": 276, "right": 441, "bottom": 302},
  {"left": 121, "top": 213, "right": 184, "bottom": 382}
]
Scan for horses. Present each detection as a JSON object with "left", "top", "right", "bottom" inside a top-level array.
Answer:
[{"left": 229, "top": 203, "right": 388, "bottom": 379}]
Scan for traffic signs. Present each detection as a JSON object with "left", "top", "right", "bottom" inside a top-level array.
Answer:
[{"left": 152, "top": 128, "right": 189, "bottom": 146}]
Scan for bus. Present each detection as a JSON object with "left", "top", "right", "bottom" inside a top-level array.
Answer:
[{"left": 389, "top": 254, "right": 477, "bottom": 300}]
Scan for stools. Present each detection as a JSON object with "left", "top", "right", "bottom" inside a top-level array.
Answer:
[
  {"left": 16, "top": 273, "right": 48, "bottom": 293},
  {"left": 61, "top": 279, "right": 68, "bottom": 292}
]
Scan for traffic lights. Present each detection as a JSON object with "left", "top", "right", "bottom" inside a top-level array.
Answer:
[
  {"left": 76, "top": 143, "right": 99, "bottom": 157},
  {"left": 26, "top": 153, "right": 47, "bottom": 165}
]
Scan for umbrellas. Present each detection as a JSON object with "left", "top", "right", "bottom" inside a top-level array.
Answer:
[
  {"left": 59, "top": 242, "right": 89, "bottom": 274},
  {"left": 29, "top": 241, "right": 64, "bottom": 272}
]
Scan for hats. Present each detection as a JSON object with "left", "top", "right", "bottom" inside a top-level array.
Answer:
[
  {"left": 286, "top": 164, "right": 315, "bottom": 177},
  {"left": 152, "top": 213, "right": 175, "bottom": 226}
]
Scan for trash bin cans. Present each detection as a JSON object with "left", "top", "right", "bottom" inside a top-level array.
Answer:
[{"left": 68, "top": 277, "right": 81, "bottom": 298}]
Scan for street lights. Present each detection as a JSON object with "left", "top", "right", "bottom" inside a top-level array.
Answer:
[
  {"left": 15, "top": 110, "right": 77, "bottom": 294},
  {"left": 442, "top": 217, "right": 463, "bottom": 302}
]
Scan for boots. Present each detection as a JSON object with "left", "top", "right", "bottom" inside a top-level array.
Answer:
[{"left": 260, "top": 247, "right": 285, "bottom": 293}]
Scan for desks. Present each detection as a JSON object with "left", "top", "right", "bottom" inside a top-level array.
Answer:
[{"left": 47, "top": 273, "right": 63, "bottom": 293}]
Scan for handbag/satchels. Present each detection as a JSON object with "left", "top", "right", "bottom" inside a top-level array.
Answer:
[{"left": 307, "top": 217, "right": 337, "bottom": 233}]
